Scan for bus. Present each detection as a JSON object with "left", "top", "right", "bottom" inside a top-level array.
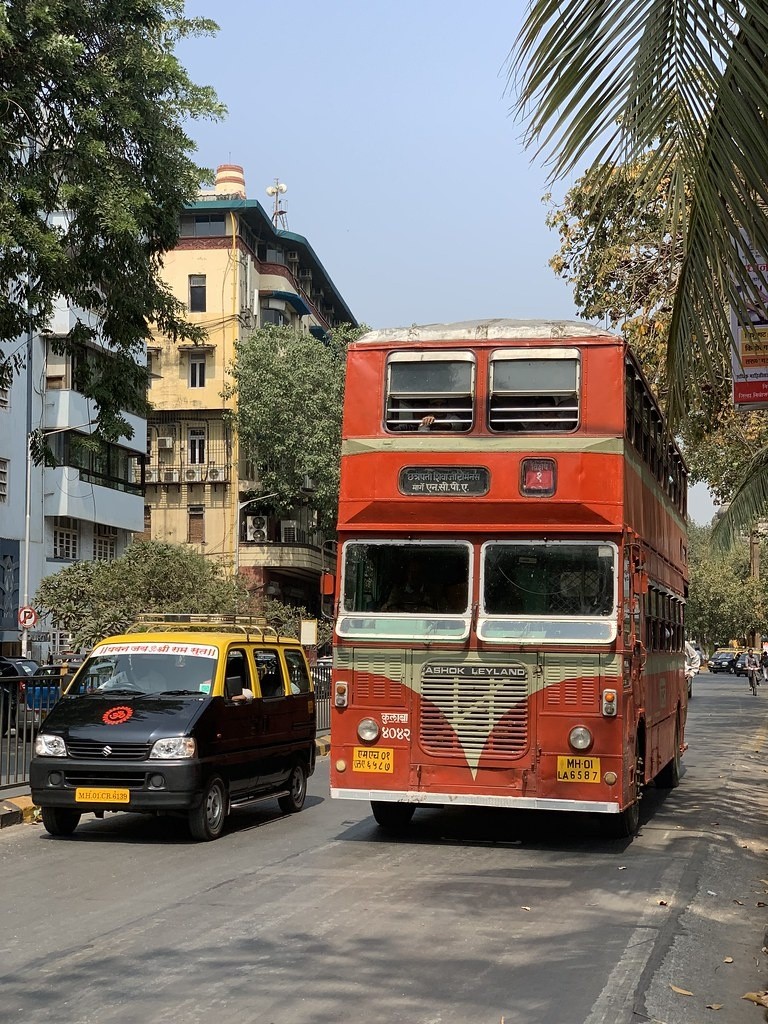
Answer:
[{"left": 316, "top": 316, "right": 690, "bottom": 845}]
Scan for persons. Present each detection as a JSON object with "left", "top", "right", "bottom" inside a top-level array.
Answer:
[
  {"left": 202, "top": 678, "right": 255, "bottom": 703},
  {"left": 96, "top": 656, "right": 167, "bottom": 693},
  {"left": 261, "top": 660, "right": 300, "bottom": 698},
  {"left": 381, "top": 557, "right": 452, "bottom": 613},
  {"left": 759, "top": 651, "right": 768, "bottom": 682},
  {"left": 524, "top": 394, "right": 578, "bottom": 431},
  {"left": 417, "top": 398, "right": 463, "bottom": 432},
  {"left": 745, "top": 649, "right": 761, "bottom": 691},
  {"left": 684, "top": 641, "right": 701, "bottom": 726}
]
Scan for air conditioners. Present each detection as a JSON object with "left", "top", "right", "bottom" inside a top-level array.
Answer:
[
  {"left": 206, "top": 468, "right": 224, "bottom": 482},
  {"left": 143, "top": 470, "right": 158, "bottom": 483},
  {"left": 184, "top": 468, "right": 202, "bottom": 481},
  {"left": 247, "top": 516, "right": 267, "bottom": 542},
  {"left": 281, "top": 520, "right": 309, "bottom": 543},
  {"left": 157, "top": 437, "right": 172, "bottom": 449},
  {"left": 161, "top": 470, "right": 179, "bottom": 482}
]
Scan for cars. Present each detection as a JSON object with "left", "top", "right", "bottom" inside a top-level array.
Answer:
[
  {"left": 0, "top": 650, "right": 115, "bottom": 747},
  {"left": 226, "top": 646, "right": 334, "bottom": 699}
]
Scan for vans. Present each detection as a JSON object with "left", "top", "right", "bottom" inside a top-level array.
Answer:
[
  {"left": 712, "top": 652, "right": 745, "bottom": 674},
  {"left": 707, "top": 648, "right": 728, "bottom": 672},
  {"left": 27, "top": 622, "right": 319, "bottom": 841},
  {"left": 736, "top": 652, "right": 761, "bottom": 678}
]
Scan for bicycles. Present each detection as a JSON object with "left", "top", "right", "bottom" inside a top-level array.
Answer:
[{"left": 747, "top": 666, "right": 760, "bottom": 696}]
